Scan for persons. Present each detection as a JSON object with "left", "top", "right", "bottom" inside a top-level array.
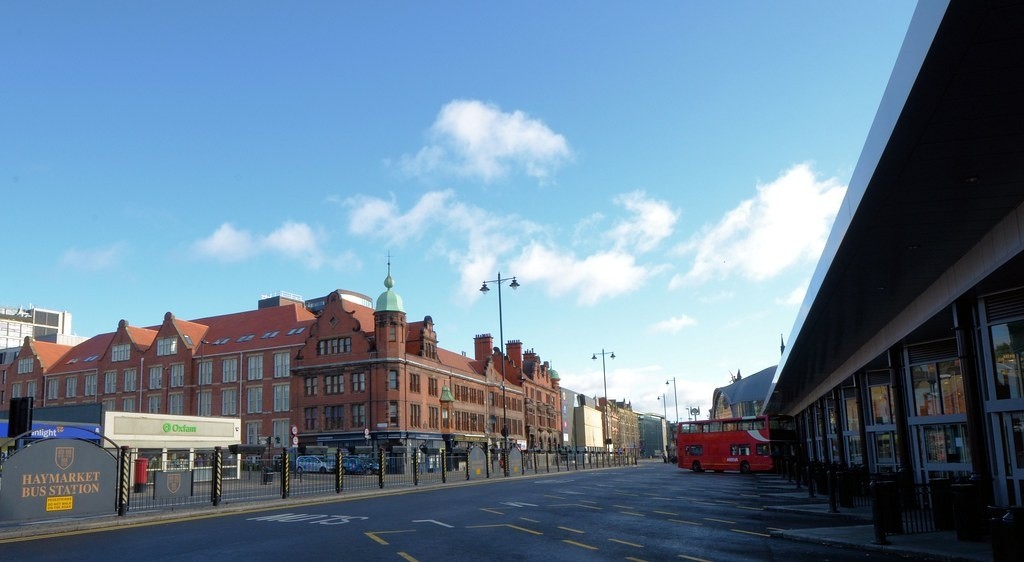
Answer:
[
  {"left": 149, "top": 456, "right": 158, "bottom": 467},
  {"left": 204, "top": 454, "right": 211, "bottom": 466},
  {"left": 670, "top": 454, "right": 675, "bottom": 465},
  {"left": 195, "top": 454, "right": 204, "bottom": 466},
  {"left": 762, "top": 445, "right": 771, "bottom": 455},
  {"left": 691, "top": 447, "right": 702, "bottom": 456},
  {"left": 733, "top": 446, "right": 739, "bottom": 456},
  {"left": 687, "top": 450, "right": 689, "bottom": 456},
  {"left": 661, "top": 452, "right": 668, "bottom": 464},
  {"left": 741, "top": 446, "right": 746, "bottom": 455}
]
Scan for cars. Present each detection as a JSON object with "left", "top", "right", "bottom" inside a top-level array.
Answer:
[
  {"left": 296, "top": 454, "right": 336, "bottom": 474},
  {"left": 325, "top": 455, "right": 380, "bottom": 477}
]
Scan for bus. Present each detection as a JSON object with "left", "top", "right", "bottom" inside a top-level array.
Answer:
[
  {"left": 676, "top": 414, "right": 799, "bottom": 476},
  {"left": 669, "top": 423, "right": 689, "bottom": 459}
]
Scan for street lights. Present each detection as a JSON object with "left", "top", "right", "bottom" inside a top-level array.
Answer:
[
  {"left": 665, "top": 377, "right": 679, "bottom": 424},
  {"left": 199, "top": 339, "right": 210, "bottom": 417},
  {"left": 480, "top": 272, "right": 520, "bottom": 479},
  {"left": 685, "top": 408, "right": 691, "bottom": 421},
  {"left": 657, "top": 393, "right": 666, "bottom": 425},
  {"left": 592, "top": 349, "right": 617, "bottom": 466}
]
